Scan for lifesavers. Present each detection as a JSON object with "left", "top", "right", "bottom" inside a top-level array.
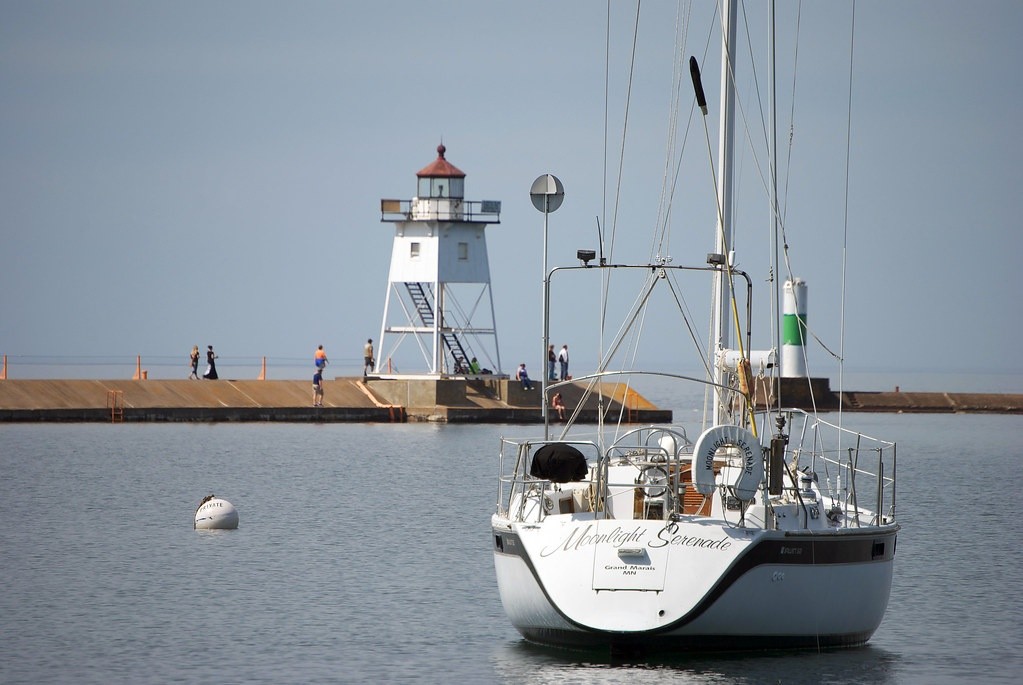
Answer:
[{"left": 689, "top": 424, "right": 764, "bottom": 501}]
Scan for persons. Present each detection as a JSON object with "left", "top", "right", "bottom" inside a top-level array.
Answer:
[
  {"left": 463, "top": 365, "right": 469, "bottom": 374},
  {"left": 469, "top": 357, "right": 479, "bottom": 375},
  {"left": 516, "top": 364, "right": 534, "bottom": 390},
  {"left": 454, "top": 356, "right": 464, "bottom": 374},
  {"left": 314, "top": 345, "right": 329, "bottom": 369},
  {"left": 364, "top": 339, "right": 375, "bottom": 376},
  {"left": 552, "top": 393, "right": 567, "bottom": 419},
  {"left": 203, "top": 346, "right": 218, "bottom": 380},
  {"left": 558, "top": 345, "right": 569, "bottom": 381},
  {"left": 188, "top": 345, "right": 200, "bottom": 380},
  {"left": 312, "top": 370, "right": 324, "bottom": 407},
  {"left": 549, "top": 345, "right": 558, "bottom": 381}
]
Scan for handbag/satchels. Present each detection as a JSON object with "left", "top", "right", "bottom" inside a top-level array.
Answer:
[{"left": 559, "top": 354, "right": 564, "bottom": 362}]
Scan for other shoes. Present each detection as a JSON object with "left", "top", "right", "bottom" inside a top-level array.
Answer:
[
  {"left": 313, "top": 404, "right": 318, "bottom": 407},
  {"left": 524, "top": 386, "right": 528, "bottom": 390},
  {"left": 559, "top": 414, "right": 562, "bottom": 419},
  {"left": 561, "top": 378, "right": 565, "bottom": 382},
  {"left": 531, "top": 387, "right": 534, "bottom": 389},
  {"left": 317, "top": 402, "right": 323, "bottom": 407}
]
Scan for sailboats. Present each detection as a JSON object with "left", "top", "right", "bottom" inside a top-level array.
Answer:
[{"left": 490, "top": 2, "right": 903, "bottom": 664}]
